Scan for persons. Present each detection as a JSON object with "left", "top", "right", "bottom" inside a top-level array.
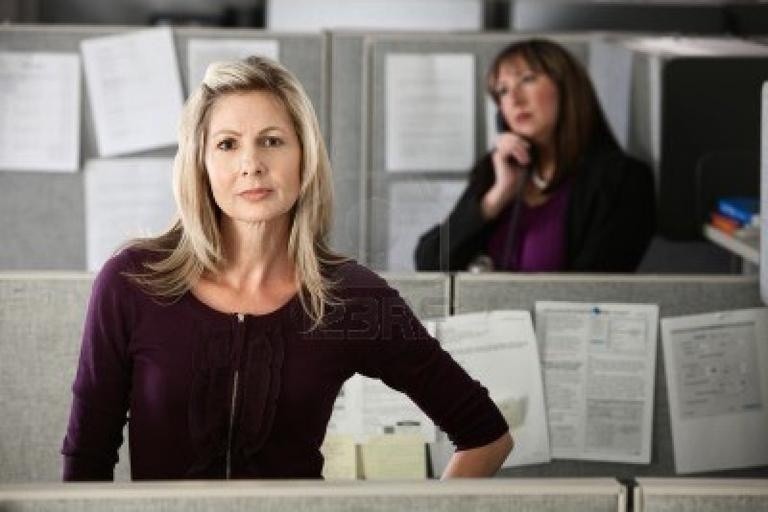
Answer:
[
  {"left": 414, "top": 37, "right": 655, "bottom": 274},
  {"left": 62, "top": 54, "right": 515, "bottom": 479}
]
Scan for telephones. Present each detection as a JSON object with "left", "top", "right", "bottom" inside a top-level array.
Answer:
[{"left": 495, "top": 106, "right": 540, "bottom": 168}]
